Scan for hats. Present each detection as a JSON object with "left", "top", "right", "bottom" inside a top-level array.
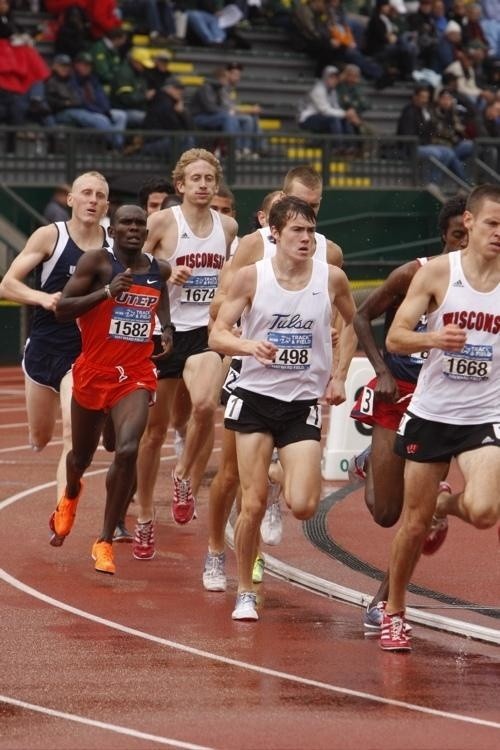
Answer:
[{"left": 53, "top": 47, "right": 244, "bottom": 71}]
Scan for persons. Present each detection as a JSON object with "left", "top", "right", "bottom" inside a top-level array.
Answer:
[
  {"left": 0, "top": 1, "right": 500, "bottom": 196},
  {"left": 206, "top": 196, "right": 357, "bottom": 620},
  {"left": 0, "top": 148, "right": 346, "bottom": 597},
  {"left": 348, "top": 195, "right": 469, "bottom": 632},
  {"left": 376, "top": 184, "right": 500, "bottom": 656}
]
